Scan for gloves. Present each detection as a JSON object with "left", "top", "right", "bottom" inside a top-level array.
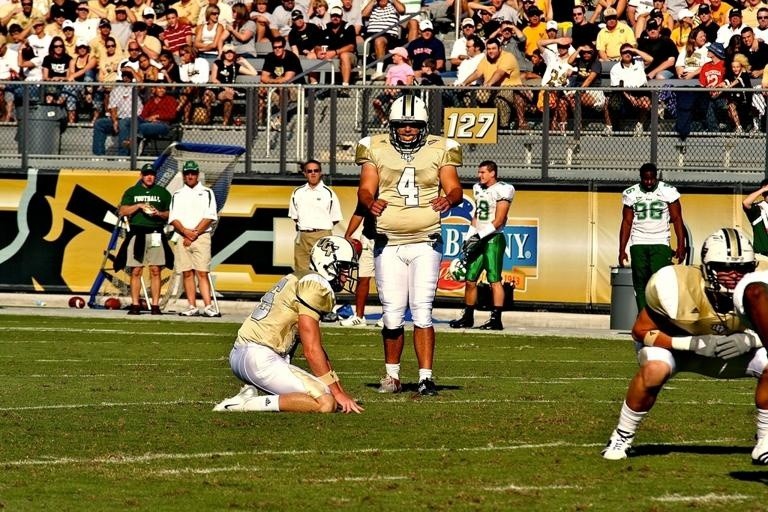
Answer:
[
  {"left": 689, "top": 335, "right": 724, "bottom": 358},
  {"left": 716, "top": 328, "right": 763, "bottom": 358},
  {"left": 463, "top": 233, "right": 480, "bottom": 257}
]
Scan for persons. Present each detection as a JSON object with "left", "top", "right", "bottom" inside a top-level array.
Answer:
[
  {"left": 601, "top": 229, "right": 768, "bottom": 465},
  {"left": 27, "top": 19, "right": 52, "bottom": 56},
  {"left": 340, "top": 188, "right": 384, "bottom": 328},
  {"left": 556, "top": 41, "right": 603, "bottom": 134},
  {"left": 596, "top": 8, "right": 639, "bottom": 62},
  {"left": 407, "top": 19, "right": 446, "bottom": 85},
  {"left": 645, "top": 0, "right": 673, "bottom": 28},
  {"left": 130, "top": 0, "right": 157, "bottom": 24},
  {"left": 1, "top": 1, "right": 24, "bottom": 29},
  {"left": 341, "top": 0, "right": 365, "bottom": 44},
  {"left": 757, "top": 7, "right": 768, "bottom": 37},
  {"left": 118, "top": 42, "right": 172, "bottom": 82},
  {"left": 468, "top": 2, "right": 496, "bottom": 36},
  {"left": 306, "top": 0, "right": 331, "bottom": 30},
  {"left": 0, "top": 0, "right": 45, "bottom": 43},
  {"left": 590, "top": 0, "right": 638, "bottom": 26},
  {"left": 176, "top": 44, "right": 210, "bottom": 125},
  {"left": 530, "top": 49, "right": 547, "bottom": 75},
  {"left": 74, "top": 3, "right": 107, "bottom": 43},
  {"left": 451, "top": 34, "right": 485, "bottom": 85},
  {"left": 537, "top": 38, "right": 578, "bottom": 88},
  {"left": 119, "top": 163, "right": 171, "bottom": 314},
  {"left": 139, "top": 54, "right": 159, "bottom": 82},
  {"left": 670, "top": 9, "right": 696, "bottom": 51},
  {"left": 355, "top": 94, "right": 463, "bottom": 395},
  {"left": 361, "top": 0, "right": 406, "bottom": 79},
  {"left": 269, "top": 0, "right": 307, "bottom": 50},
  {"left": 450, "top": 160, "right": 516, "bottom": 332},
  {"left": 261, "top": 36, "right": 310, "bottom": 112},
  {"left": 742, "top": 179, "right": 768, "bottom": 272},
  {"left": 42, "top": 37, "right": 73, "bottom": 105},
  {"left": 217, "top": 3, "right": 257, "bottom": 60},
  {"left": 129, "top": 7, "right": 164, "bottom": 54},
  {"left": 9, "top": 24, "right": 23, "bottom": 80},
  {"left": 111, "top": 5, "right": 136, "bottom": 48},
  {"left": 742, "top": 1, "right": 767, "bottom": 29},
  {"left": 49, "top": 1, "right": 79, "bottom": 20},
  {"left": 199, "top": 0, "right": 234, "bottom": 27},
  {"left": 56, "top": 41, "right": 98, "bottom": 123},
  {"left": 124, "top": 21, "right": 162, "bottom": 61},
  {"left": 288, "top": 160, "right": 343, "bottom": 322},
  {"left": 491, "top": 0, "right": 521, "bottom": 25},
  {"left": 546, "top": 20, "right": 560, "bottom": 37},
  {"left": 711, "top": 0, "right": 732, "bottom": 25},
  {"left": 638, "top": 19, "right": 679, "bottom": 121},
  {"left": 167, "top": 161, "right": 221, "bottom": 318},
  {"left": 418, "top": 58, "right": 445, "bottom": 86},
  {"left": 211, "top": 235, "right": 363, "bottom": 415},
  {"left": 723, "top": 53, "right": 754, "bottom": 131},
  {"left": 761, "top": 64, "right": 768, "bottom": 100},
  {"left": 168, "top": 0, "right": 201, "bottom": 24},
  {"left": 373, "top": 47, "right": 413, "bottom": 132},
  {"left": 521, "top": 0, "right": 536, "bottom": 17},
  {"left": 90, "top": 37, "right": 122, "bottom": 123},
  {"left": 716, "top": 7, "right": 751, "bottom": 48},
  {"left": 204, "top": 44, "right": 258, "bottom": 130},
  {"left": 650, "top": 8, "right": 672, "bottom": 38},
  {"left": 731, "top": 272, "right": 768, "bottom": 353},
  {"left": 674, "top": 29, "right": 713, "bottom": 129},
  {"left": 450, "top": 18, "right": 484, "bottom": 64},
  {"left": 93, "top": 67, "right": 144, "bottom": 156},
  {"left": 306, "top": 6, "right": 358, "bottom": 94},
  {"left": 572, "top": 5, "right": 600, "bottom": 49},
  {"left": 249, "top": 0, "right": 269, "bottom": 42},
  {"left": 490, "top": 21, "right": 532, "bottom": 71},
  {"left": 195, "top": 4, "right": 224, "bottom": 58},
  {"left": 61, "top": 19, "right": 78, "bottom": 56},
  {"left": 608, "top": 42, "right": 653, "bottom": 133},
  {"left": 724, "top": 34, "right": 743, "bottom": 83},
  {"left": 288, "top": 9, "right": 322, "bottom": 59},
  {"left": 89, "top": 18, "right": 123, "bottom": 57},
  {"left": 124, "top": 83, "right": 178, "bottom": 155},
  {"left": 521, "top": 6, "right": 548, "bottom": 58},
  {"left": 617, "top": 163, "right": 676, "bottom": 314},
  {"left": 158, "top": 8, "right": 192, "bottom": 55},
  {"left": 688, "top": 4, "right": 719, "bottom": 51},
  {"left": 699, "top": 43, "right": 728, "bottom": 129},
  {"left": 43, "top": 7, "right": 67, "bottom": 36},
  {"left": 0, "top": 26, "right": 21, "bottom": 80},
  {"left": 17, "top": 40, "right": 44, "bottom": 82},
  {"left": 741, "top": 27, "right": 767, "bottom": 87},
  {"left": 158, "top": 50, "right": 179, "bottom": 98},
  {"left": 87, "top": 1, "right": 116, "bottom": 20},
  {"left": 460, "top": 39, "right": 520, "bottom": 131}
]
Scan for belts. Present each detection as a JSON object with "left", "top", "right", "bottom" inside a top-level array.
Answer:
[{"left": 301, "top": 230, "right": 322, "bottom": 233}]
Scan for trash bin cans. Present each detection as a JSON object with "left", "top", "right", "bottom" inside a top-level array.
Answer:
[
  {"left": 14, "top": 100, "right": 66, "bottom": 154},
  {"left": 610, "top": 265, "right": 639, "bottom": 329}
]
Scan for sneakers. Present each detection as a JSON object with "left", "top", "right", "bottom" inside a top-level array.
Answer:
[
  {"left": 152, "top": 305, "right": 162, "bottom": 316},
  {"left": 203, "top": 304, "right": 221, "bottom": 319},
  {"left": 417, "top": 379, "right": 438, "bottom": 398},
  {"left": 180, "top": 305, "right": 200, "bottom": 316},
  {"left": 601, "top": 429, "right": 636, "bottom": 459},
  {"left": 479, "top": 316, "right": 504, "bottom": 330},
  {"left": 338, "top": 315, "right": 367, "bottom": 328},
  {"left": 212, "top": 386, "right": 258, "bottom": 412},
  {"left": 325, "top": 312, "right": 338, "bottom": 322},
  {"left": 375, "top": 318, "right": 385, "bottom": 328},
  {"left": 752, "top": 444, "right": 768, "bottom": 464},
  {"left": 127, "top": 305, "right": 141, "bottom": 316},
  {"left": 450, "top": 316, "right": 475, "bottom": 330},
  {"left": 377, "top": 375, "right": 402, "bottom": 394}
]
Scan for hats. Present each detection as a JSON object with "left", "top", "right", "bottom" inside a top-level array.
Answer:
[
  {"left": 646, "top": 20, "right": 659, "bottom": 30},
  {"left": 291, "top": 10, "right": 304, "bottom": 20},
  {"left": 603, "top": 9, "right": 618, "bottom": 17},
  {"left": 419, "top": 21, "right": 434, "bottom": 32},
  {"left": 546, "top": 20, "right": 558, "bottom": 31},
  {"left": 462, "top": 18, "right": 475, "bottom": 27},
  {"left": 182, "top": 161, "right": 199, "bottom": 172},
  {"left": 62, "top": 21, "right": 74, "bottom": 28},
  {"left": 389, "top": 47, "right": 409, "bottom": 59},
  {"left": 143, "top": 8, "right": 155, "bottom": 15},
  {"left": 729, "top": 9, "right": 742, "bottom": 16},
  {"left": 77, "top": 40, "right": 88, "bottom": 46},
  {"left": 526, "top": 6, "right": 543, "bottom": 16},
  {"left": 140, "top": 164, "right": 157, "bottom": 175},
  {"left": 650, "top": 8, "right": 663, "bottom": 17},
  {"left": 698, "top": 4, "right": 710, "bottom": 13},
  {"left": 330, "top": 6, "right": 344, "bottom": 16},
  {"left": 707, "top": 43, "right": 726, "bottom": 60}
]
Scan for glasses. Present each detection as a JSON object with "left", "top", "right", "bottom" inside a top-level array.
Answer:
[
  {"left": 22, "top": 2, "right": 32, "bottom": 6},
  {"left": 129, "top": 48, "right": 139, "bottom": 51},
  {"left": 53, "top": 45, "right": 64, "bottom": 48},
  {"left": 106, "top": 45, "right": 115, "bottom": 48},
  {"left": 180, "top": 52, "right": 187, "bottom": 57},
  {"left": 573, "top": 12, "right": 583, "bottom": 17},
  {"left": 306, "top": 169, "right": 320, "bottom": 174},
  {"left": 211, "top": 11, "right": 220, "bottom": 16},
  {"left": 757, "top": 16, "right": 768, "bottom": 20},
  {"left": 273, "top": 45, "right": 283, "bottom": 48}
]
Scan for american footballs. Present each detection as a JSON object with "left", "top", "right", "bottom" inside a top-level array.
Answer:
[
  {"left": 104, "top": 298, "right": 120, "bottom": 311},
  {"left": 68, "top": 297, "right": 84, "bottom": 309}
]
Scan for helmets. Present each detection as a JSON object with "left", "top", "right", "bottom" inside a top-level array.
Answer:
[
  {"left": 702, "top": 228, "right": 757, "bottom": 285},
  {"left": 450, "top": 258, "right": 467, "bottom": 281},
  {"left": 311, "top": 235, "right": 360, "bottom": 294},
  {"left": 389, "top": 95, "right": 428, "bottom": 149}
]
